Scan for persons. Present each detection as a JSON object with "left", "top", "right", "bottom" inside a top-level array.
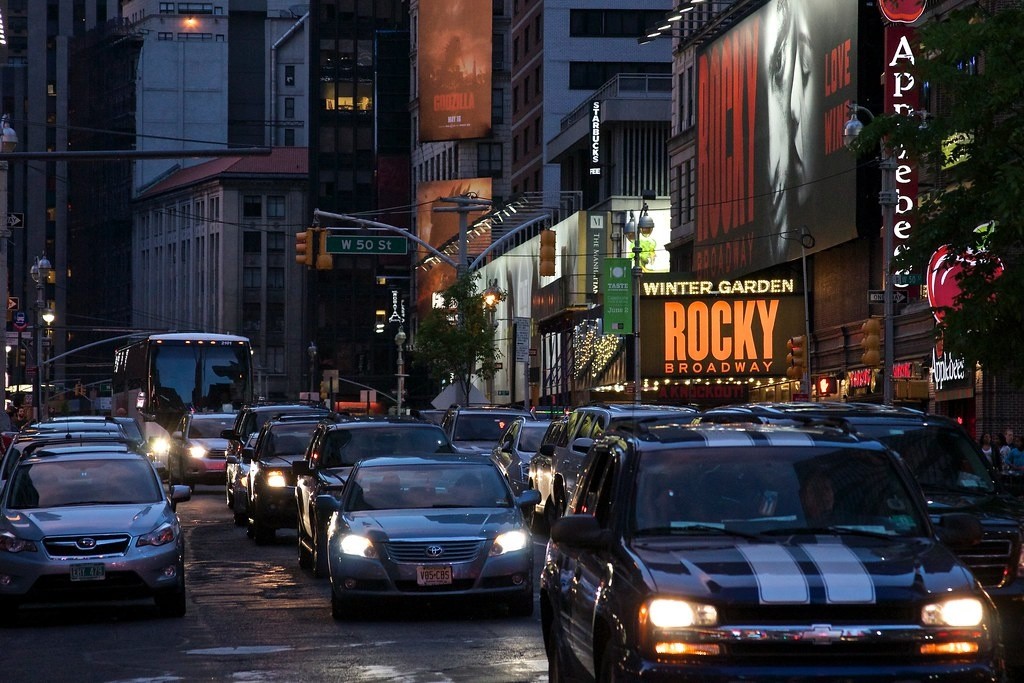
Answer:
[
  {"left": 5, "top": 406, "right": 30, "bottom": 431},
  {"left": 796, "top": 465, "right": 835, "bottom": 527},
  {"left": 758, "top": 18, "right": 849, "bottom": 262},
  {"left": 979, "top": 430, "right": 1024, "bottom": 502}
]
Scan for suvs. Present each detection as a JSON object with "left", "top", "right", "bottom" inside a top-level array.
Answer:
[
  {"left": 219, "top": 403, "right": 319, "bottom": 528},
  {"left": 696, "top": 399, "right": 1023, "bottom": 658},
  {"left": 529, "top": 402, "right": 708, "bottom": 530},
  {"left": 292, "top": 407, "right": 459, "bottom": 579},
  {"left": 539, "top": 421, "right": 1009, "bottom": 683},
  {"left": 442, "top": 402, "right": 536, "bottom": 463}
]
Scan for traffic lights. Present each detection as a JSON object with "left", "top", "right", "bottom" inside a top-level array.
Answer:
[
  {"left": 15, "top": 345, "right": 27, "bottom": 368},
  {"left": 80, "top": 383, "right": 87, "bottom": 396},
  {"left": 296, "top": 227, "right": 313, "bottom": 269}
]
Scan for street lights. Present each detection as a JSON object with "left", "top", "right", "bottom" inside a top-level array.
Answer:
[
  {"left": 839, "top": 102, "right": 937, "bottom": 401},
  {"left": 27, "top": 251, "right": 54, "bottom": 420},
  {"left": 621, "top": 200, "right": 655, "bottom": 400},
  {"left": 392, "top": 322, "right": 409, "bottom": 417},
  {"left": 306, "top": 339, "right": 318, "bottom": 393},
  {"left": 487, "top": 278, "right": 504, "bottom": 407}
]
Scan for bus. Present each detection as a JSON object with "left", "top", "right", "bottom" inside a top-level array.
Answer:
[{"left": 109, "top": 331, "right": 255, "bottom": 477}]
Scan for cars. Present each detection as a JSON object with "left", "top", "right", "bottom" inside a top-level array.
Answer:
[
  {"left": 168, "top": 408, "right": 242, "bottom": 499},
  {"left": 492, "top": 414, "right": 559, "bottom": 499},
  {"left": 316, "top": 455, "right": 546, "bottom": 620},
  {"left": 240, "top": 407, "right": 340, "bottom": 542},
  {"left": 0, "top": 415, "right": 194, "bottom": 621}
]
